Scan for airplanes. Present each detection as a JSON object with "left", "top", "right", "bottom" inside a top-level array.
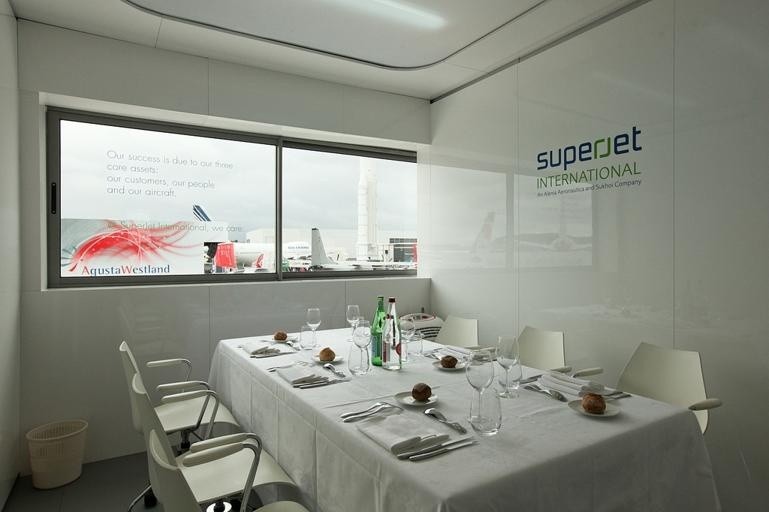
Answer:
[{"left": 191, "top": 204, "right": 417, "bottom": 273}]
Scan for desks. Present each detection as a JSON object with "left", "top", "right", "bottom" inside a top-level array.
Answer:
[{"left": 207, "top": 324, "right": 723, "bottom": 512}]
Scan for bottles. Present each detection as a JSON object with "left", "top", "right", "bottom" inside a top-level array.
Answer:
[
  {"left": 370, "top": 296, "right": 390, "bottom": 367},
  {"left": 381, "top": 297, "right": 402, "bottom": 371}
]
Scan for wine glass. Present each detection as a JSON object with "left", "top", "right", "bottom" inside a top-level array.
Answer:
[
  {"left": 307, "top": 307, "right": 322, "bottom": 348},
  {"left": 353, "top": 320, "right": 372, "bottom": 371},
  {"left": 398, "top": 317, "right": 415, "bottom": 365},
  {"left": 495, "top": 334, "right": 521, "bottom": 399},
  {"left": 346, "top": 304, "right": 360, "bottom": 343}
]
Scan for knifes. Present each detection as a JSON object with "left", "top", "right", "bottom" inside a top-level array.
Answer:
[
  {"left": 525, "top": 384, "right": 567, "bottom": 403},
  {"left": 396, "top": 437, "right": 474, "bottom": 462}
]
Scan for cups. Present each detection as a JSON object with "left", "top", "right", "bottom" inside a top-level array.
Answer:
[
  {"left": 299, "top": 324, "right": 317, "bottom": 350},
  {"left": 498, "top": 359, "right": 523, "bottom": 390},
  {"left": 348, "top": 344, "right": 369, "bottom": 377},
  {"left": 405, "top": 330, "right": 422, "bottom": 355}
]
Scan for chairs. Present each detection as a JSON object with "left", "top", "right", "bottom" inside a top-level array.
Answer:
[
  {"left": 145, "top": 429, "right": 308, "bottom": 512},
  {"left": 474, "top": 322, "right": 571, "bottom": 373},
  {"left": 125, "top": 372, "right": 299, "bottom": 509},
  {"left": 119, "top": 339, "right": 240, "bottom": 453},
  {"left": 571, "top": 339, "right": 722, "bottom": 437},
  {"left": 431, "top": 314, "right": 499, "bottom": 353}
]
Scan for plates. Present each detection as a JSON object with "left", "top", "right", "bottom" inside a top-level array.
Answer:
[
  {"left": 567, "top": 399, "right": 621, "bottom": 419},
  {"left": 432, "top": 360, "right": 465, "bottom": 371},
  {"left": 394, "top": 389, "right": 438, "bottom": 407},
  {"left": 312, "top": 354, "right": 344, "bottom": 364}
]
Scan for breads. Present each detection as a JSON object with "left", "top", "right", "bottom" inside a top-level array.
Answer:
[
  {"left": 411, "top": 382, "right": 431, "bottom": 401},
  {"left": 274, "top": 331, "right": 287, "bottom": 341},
  {"left": 440, "top": 355, "right": 457, "bottom": 368},
  {"left": 319, "top": 347, "right": 335, "bottom": 361},
  {"left": 581, "top": 392, "right": 606, "bottom": 414}
]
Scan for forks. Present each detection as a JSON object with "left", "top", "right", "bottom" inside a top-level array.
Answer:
[{"left": 340, "top": 400, "right": 403, "bottom": 422}]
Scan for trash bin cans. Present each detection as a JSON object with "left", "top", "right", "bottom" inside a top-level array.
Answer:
[{"left": 26, "top": 419, "right": 88, "bottom": 490}]
